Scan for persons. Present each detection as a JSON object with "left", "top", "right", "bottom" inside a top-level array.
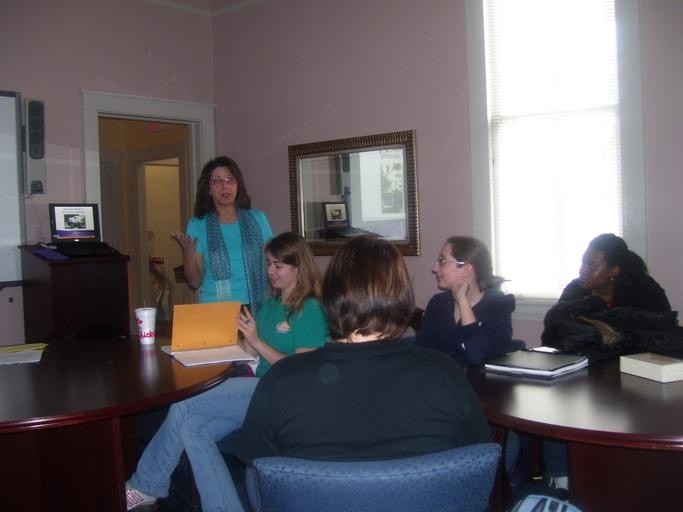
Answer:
[
  {"left": 418, "top": 235, "right": 523, "bottom": 502},
  {"left": 541, "top": 234, "right": 681, "bottom": 504},
  {"left": 171, "top": 156, "right": 275, "bottom": 320},
  {"left": 239, "top": 237, "right": 494, "bottom": 466},
  {"left": 126, "top": 230, "right": 328, "bottom": 512}
]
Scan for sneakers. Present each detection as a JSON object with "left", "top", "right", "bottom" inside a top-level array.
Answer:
[{"left": 126, "top": 482, "right": 157, "bottom": 510}]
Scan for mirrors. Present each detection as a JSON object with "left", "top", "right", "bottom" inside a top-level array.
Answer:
[{"left": 287, "top": 128, "right": 423, "bottom": 257}]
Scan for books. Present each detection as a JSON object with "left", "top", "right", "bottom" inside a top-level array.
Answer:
[
  {"left": 483, "top": 347, "right": 589, "bottom": 379},
  {"left": 160, "top": 345, "right": 255, "bottom": 366}
]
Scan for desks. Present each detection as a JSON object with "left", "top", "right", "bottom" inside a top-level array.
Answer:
[
  {"left": 0, "top": 327, "right": 233, "bottom": 512},
  {"left": 463, "top": 350, "right": 683, "bottom": 512}
]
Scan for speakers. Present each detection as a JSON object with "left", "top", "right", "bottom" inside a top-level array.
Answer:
[
  {"left": 24, "top": 96, "right": 48, "bottom": 194},
  {"left": 339, "top": 152, "right": 353, "bottom": 197}
]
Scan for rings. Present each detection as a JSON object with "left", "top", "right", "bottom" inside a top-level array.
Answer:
[{"left": 245, "top": 320, "right": 251, "bottom": 324}]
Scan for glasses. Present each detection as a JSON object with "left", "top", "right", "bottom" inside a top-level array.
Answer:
[
  {"left": 437, "top": 257, "right": 465, "bottom": 267},
  {"left": 208, "top": 176, "right": 237, "bottom": 186}
]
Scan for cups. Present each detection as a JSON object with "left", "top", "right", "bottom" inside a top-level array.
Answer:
[{"left": 135, "top": 306, "right": 157, "bottom": 345}]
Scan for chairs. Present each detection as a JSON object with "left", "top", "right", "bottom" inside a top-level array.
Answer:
[{"left": 240, "top": 440, "right": 503, "bottom": 512}]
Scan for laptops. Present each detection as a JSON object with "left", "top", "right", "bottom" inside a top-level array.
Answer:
[
  {"left": 50, "top": 204, "right": 120, "bottom": 257},
  {"left": 324, "top": 201, "right": 349, "bottom": 231}
]
